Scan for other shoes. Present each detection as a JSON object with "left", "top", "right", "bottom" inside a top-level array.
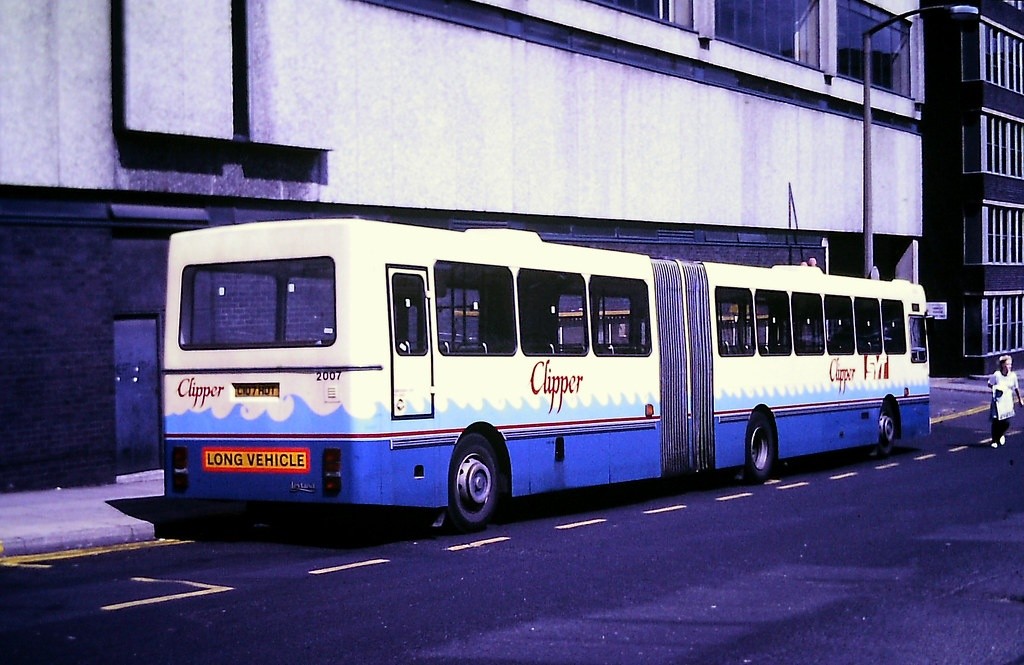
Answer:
[
  {"left": 991, "top": 442, "right": 998, "bottom": 448},
  {"left": 1000, "top": 435, "right": 1006, "bottom": 446}
]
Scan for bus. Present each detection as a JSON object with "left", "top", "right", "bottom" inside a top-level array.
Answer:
[{"left": 154, "top": 216, "right": 933, "bottom": 538}]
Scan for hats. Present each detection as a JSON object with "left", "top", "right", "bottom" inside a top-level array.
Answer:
[{"left": 999, "top": 355, "right": 1012, "bottom": 361}]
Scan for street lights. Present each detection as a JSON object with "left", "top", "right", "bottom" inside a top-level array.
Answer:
[{"left": 858, "top": 5, "right": 981, "bottom": 282}]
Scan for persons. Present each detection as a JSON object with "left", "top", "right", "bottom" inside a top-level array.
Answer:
[{"left": 987, "top": 355, "right": 1024, "bottom": 448}]
